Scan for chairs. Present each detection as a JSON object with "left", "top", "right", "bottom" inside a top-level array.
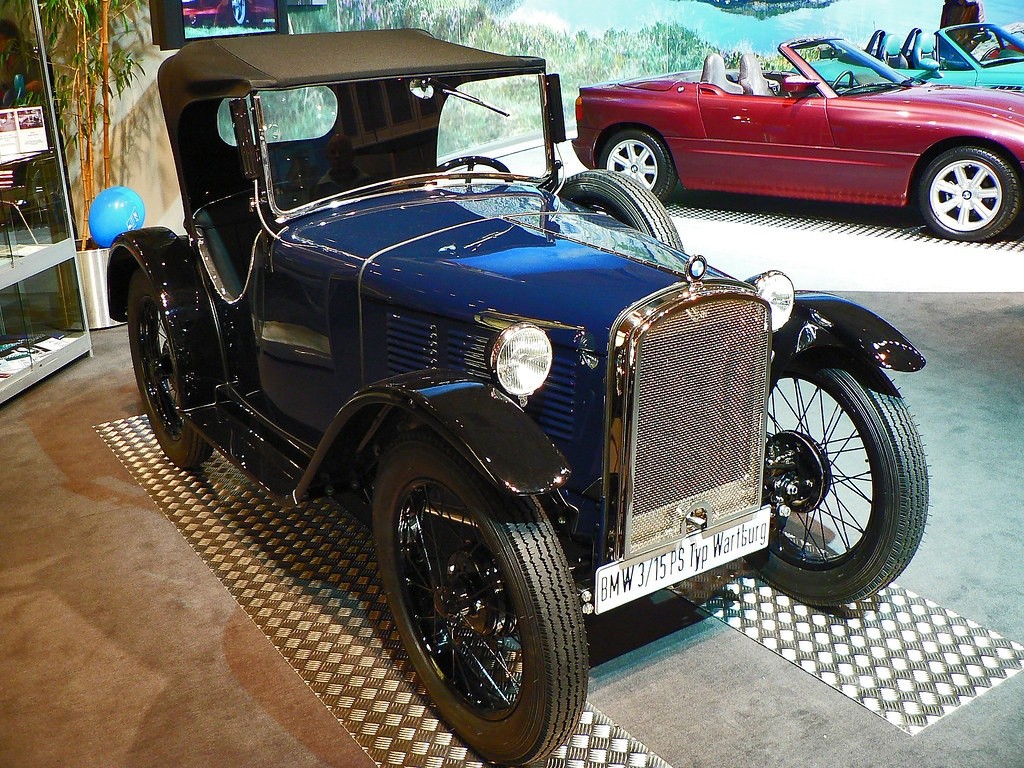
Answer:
[
  {"left": 738, "top": 53, "right": 778, "bottom": 96},
  {"left": 913, "top": 31, "right": 941, "bottom": 72},
  {"left": 192, "top": 180, "right": 314, "bottom": 305},
  {"left": 699, "top": 54, "right": 744, "bottom": 95},
  {"left": 879, "top": 33, "right": 909, "bottom": 70}
]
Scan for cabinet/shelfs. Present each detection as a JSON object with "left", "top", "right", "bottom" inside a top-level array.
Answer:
[{"left": 0, "top": 0, "right": 95, "bottom": 408}]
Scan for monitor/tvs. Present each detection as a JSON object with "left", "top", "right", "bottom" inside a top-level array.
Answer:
[{"left": 148, "top": 0, "right": 289, "bottom": 51}]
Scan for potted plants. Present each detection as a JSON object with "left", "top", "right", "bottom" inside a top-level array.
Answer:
[{"left": 0, "top": 0, "right": 147, "bottom": 333}]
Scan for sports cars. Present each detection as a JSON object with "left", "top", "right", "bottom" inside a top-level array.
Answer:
[
  {"left": 571, "top": 35, "right": 1023, "bottom": 245},
  {"left": 789, "top": 22, "right": 1024, "bottom": 96}
]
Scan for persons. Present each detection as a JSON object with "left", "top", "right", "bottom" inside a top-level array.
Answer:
[
  {"left": 940, "top": 0, "right": 986, "bottom": 60},
  {"left": 0, "top": 17, "right": 63, "bottom": 226},
  {"left": 313, "top": 133, "right": 378, "bottom": 201}
]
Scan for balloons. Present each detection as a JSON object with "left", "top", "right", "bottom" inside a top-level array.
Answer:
[{"left": 87, "top": 185, "right": 146, "bottom": 248}]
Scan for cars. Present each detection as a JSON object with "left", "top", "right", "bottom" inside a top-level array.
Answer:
[{"left": 102, "top": 27, "right": 932, "bottom": 768}]
